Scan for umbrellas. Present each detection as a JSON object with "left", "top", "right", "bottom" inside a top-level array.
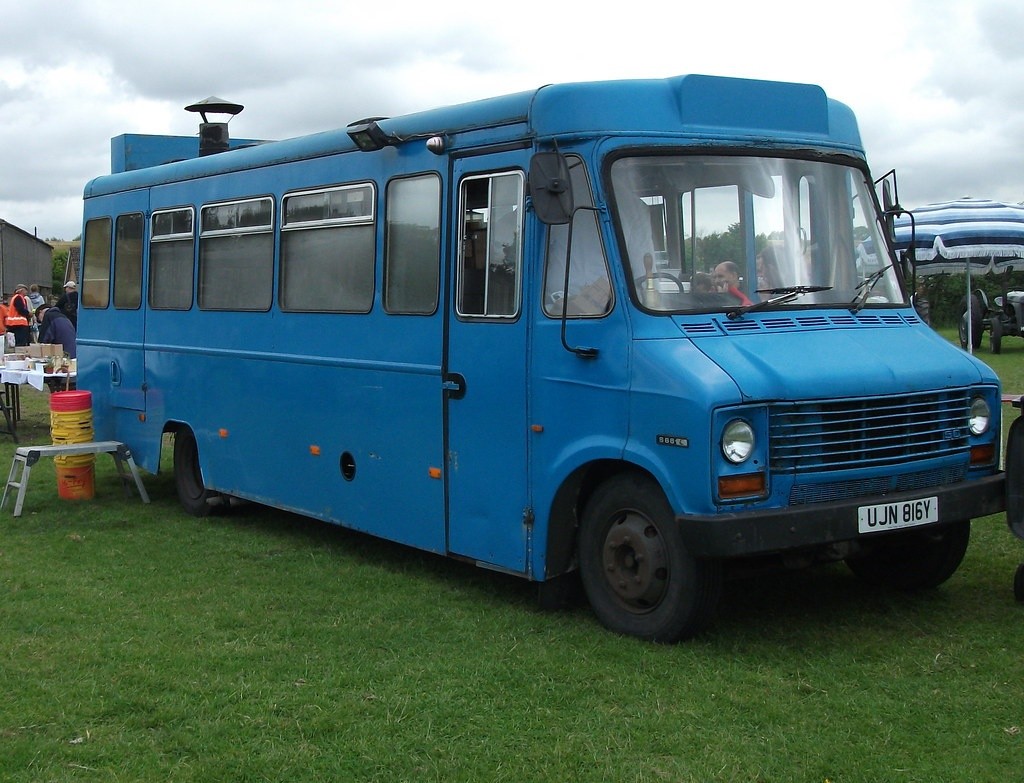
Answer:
[{"left": 858, "top": 195, "right": 1023, "bottom": 356}]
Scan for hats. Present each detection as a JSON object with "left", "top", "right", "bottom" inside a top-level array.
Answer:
[
  {"left": 62, "top": 281, "right": 76, "bottom": 289},
  {"left": 34, "top": 304, "right": 53, "bottom": 324},
  {"left": 14, "top": 284, "right": 29, "bottom": 292}
]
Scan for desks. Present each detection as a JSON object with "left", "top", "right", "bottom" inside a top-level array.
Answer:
[{"left": 0, "top": 366, "right": 77, "bottom": 432}]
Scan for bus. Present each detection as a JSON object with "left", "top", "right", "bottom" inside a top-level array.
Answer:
[{"left": 75, "top": 70, "right": 1012, "bottom": 640}]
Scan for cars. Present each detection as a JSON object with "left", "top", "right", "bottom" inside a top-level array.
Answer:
[{"left": 957, "top": 264, "right": 1024, "bottom": 355}]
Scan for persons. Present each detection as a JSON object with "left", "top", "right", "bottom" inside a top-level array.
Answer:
[
  {"left": 910, "top": 285, "right": 929, "bottom": 325},
  {"left": 999, "top": 304, "right": 1017, "bottom": 335},
  {"left": 34, "top": 303, "right": 76, "bottom": 394},
  {"left": 0, "top": 304, "right": 9, "bottom": 356},
  {"left": 712, "top": 260, "right": 742, "bottom": 293},
  {"left": 28, "top": 284, "right": 45, "bottom": 343},
  {"left": 55, "top": 281, "right": 77, "bottom": 335},
  {"left": 24, "top": 295, "right": 34, "bottom": 344},
  {"left": 8, "top": 283, "right": 33, "bottom": 346},
  {"left": 689, "top": 271, "right": 728, "bottom": 297}
]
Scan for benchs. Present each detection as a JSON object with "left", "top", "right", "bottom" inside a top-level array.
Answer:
[{"left": 0, "top": 440, "right": 151, "bottom": 517}]
